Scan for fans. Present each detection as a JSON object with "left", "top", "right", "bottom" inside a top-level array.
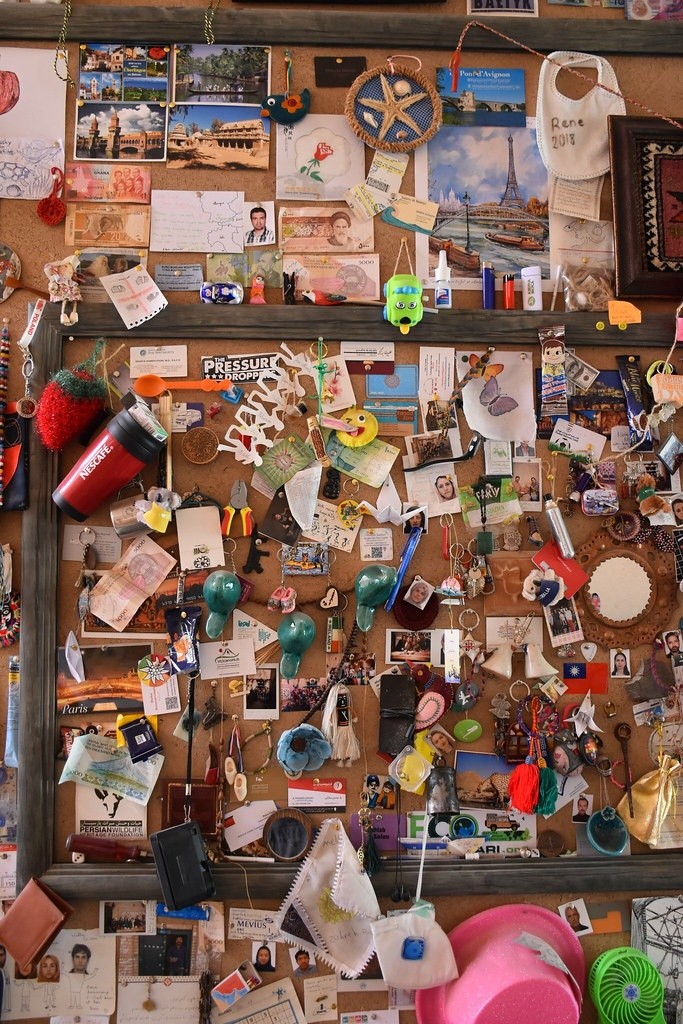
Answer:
[{"left": 588, "top": 946, "right": 667, "bottom": 1024}]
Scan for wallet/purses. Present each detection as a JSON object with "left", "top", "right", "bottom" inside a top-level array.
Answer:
[{"left": 0, "top": 875, "right": 76, "bottom": 972}]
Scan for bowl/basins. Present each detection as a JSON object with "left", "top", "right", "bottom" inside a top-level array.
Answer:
[{"left": 262, "top": 807, "right": 313, "bottom": 862}]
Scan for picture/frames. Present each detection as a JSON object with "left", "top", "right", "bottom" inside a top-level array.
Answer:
[
  {"left": 607, "top": 114, "right": 683, "bottom": 301},
  {"left": 16, "top": 300, "right": 683, "bottom": 899}
]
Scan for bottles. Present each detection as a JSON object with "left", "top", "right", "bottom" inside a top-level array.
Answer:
[{"left": 434, "top": 250, "right": 452, "bottom": 309}]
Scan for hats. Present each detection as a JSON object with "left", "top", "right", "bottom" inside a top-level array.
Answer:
[{"left": 414, "top": 904, "right": 585, "bottom": 1024}]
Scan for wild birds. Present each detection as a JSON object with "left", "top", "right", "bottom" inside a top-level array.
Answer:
[
  {"left": 187, "top": 79, "right": 245, "bottom": 92},
  {"left": 195, "top": 93, "right": 244, "bottom": 103}
]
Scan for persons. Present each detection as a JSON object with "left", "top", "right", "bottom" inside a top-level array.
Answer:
[
  {"left": 245, "top": 207, "right": 274, "bottom": 243},
  {"left": 0, "top": 944, "right": 91, "bottom": 983},
  {"left": 167, "top": 936, "right": 188, "bottom": 976},
  {"left": 592, "top": 593, "right": 600, "bottom": 611},
  {"left": 573, "top": 797, "right": 590, "bottom": 822},
  {"left": 330, "top": 659, "right": 375, "bottom": 685},
  {"left": 565, "top": 906, "right": 588, "bottom": 932},
  {"left": 434, "top": 474, "right": 458, "bottom": 502},
  {"left": 251, "top": 250, "right": 280, "bottom": 287},
  {"left": 327, "top": 211, "right": 352, "bottom": 246},
  {"left": 293, "top": 950, "right": 317, "bottom": 976},
  {"left": 284, "top": 686, "right": 327, "bottom": 710},
  {"left": 406, "top": 583, "right": 429, "bottom": 610},
  {"left": 672, "top": 499, "right": 683, "bottom": 526},
  {"left": 113, "top": 168, "right": 144, "bottom": 198},
  {"left": 253, "top": 945, "right": 276, "bottom": 971},
  {"left": 404, "top": 506, "right": 426, "bottom": 533},
  {"left": 430, "top": 731, "right": 457, "bottom": 756},
  {"left": 516, "top": 441, "right": 534, "bottom": 456},
  {"left": 512, "top": 476, "right": 539, "bottom": 501},
  {"left": 118, "top": 915, "right": 146, "bottom": 929},
  {"left": 552, "top": 607, "right": 576, "bottom": 636},
  {"left": 665, "top": 632, "right": 683, "bottom": 666},
  {"left": 393, "top": 634, "right": 430, "bottom": 651},
  {"left": 612, "top": 653, "right": 630, "bottom": 676}
]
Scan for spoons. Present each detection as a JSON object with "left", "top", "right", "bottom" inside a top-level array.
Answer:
[{"left": 133, "top": 375, "right": 233, "bottom": 396}]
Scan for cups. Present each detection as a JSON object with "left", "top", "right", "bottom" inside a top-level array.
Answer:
[
  {"left": 109, "top": 481, "right": 158, "bottom": 541},
  {"left": 51, "top": 409, "right": 167, "bottom": 524}
]
